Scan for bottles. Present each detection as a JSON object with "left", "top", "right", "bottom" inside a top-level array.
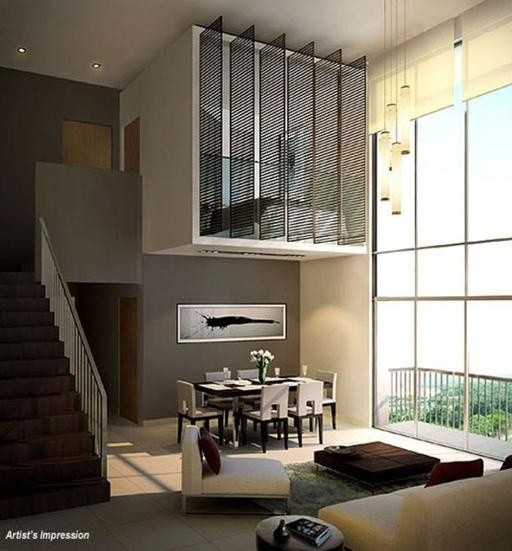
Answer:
[{"left": 274, "top": 520, "right": 291, "bottom": 548}]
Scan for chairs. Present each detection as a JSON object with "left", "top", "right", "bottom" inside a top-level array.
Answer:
[
  {"left": 177, "top": 368, "right": 337, "bottom": 453},
  {"left": 181, "top": 424, "right": 291, "bottom": 516}
]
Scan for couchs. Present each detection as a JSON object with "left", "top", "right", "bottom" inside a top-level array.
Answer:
[{"left": 317, "top": 468, "right": 512, "bottom": 551}]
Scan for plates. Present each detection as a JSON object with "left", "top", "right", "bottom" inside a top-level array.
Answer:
[
  {"left": 323, "top": 447, "right": 361, "bottom": 457},
  {"left": 198, "top": 378, "right": 312, "bottom": 392}
]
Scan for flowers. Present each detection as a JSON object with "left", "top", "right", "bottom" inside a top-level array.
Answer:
[{"left": 248, "top": 350, "right": 275, "bottom": 379}]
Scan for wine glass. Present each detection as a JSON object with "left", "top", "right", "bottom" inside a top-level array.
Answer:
[
  {"left": 302, "top": 364, "right": 308, "bottom": 381},
  {"left": 275, "top": 367, "right": 280, "bottom": 381}
]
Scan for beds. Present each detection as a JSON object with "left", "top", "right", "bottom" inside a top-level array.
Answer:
[{"left": 200, "top": 202, "right": 348, "bottom": 242}]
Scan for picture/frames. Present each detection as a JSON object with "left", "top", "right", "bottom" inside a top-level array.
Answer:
[{"left": 177, "top": 303, "right": 287, "bottom": 343}]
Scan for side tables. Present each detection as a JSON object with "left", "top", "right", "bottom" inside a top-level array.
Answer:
[{"left": 254, "top": 512, "right": 345, "bottom": 551}]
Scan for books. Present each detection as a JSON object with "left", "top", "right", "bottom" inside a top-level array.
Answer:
[{"left": 285, "top": 518, "right": 334, "bottom": 548}]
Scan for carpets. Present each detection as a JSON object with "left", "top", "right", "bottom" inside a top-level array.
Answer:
[{"left": 250, "top": 461, "right": 435, "bottom": 518}]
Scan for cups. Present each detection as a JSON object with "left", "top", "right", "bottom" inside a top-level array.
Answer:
[{"left": 223, "top": 368, "right": 229, "bottom": 381}]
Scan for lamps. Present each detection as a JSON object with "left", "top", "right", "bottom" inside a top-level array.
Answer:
[{"left": 379, "top": 0, "right": 410, "bottom": 215}]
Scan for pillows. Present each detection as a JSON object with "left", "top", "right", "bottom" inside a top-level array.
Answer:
[
  {"left": 425, "top": 459, "right": 483, "bottom": 488},
  {"left": 199, "top": 426, "right": 221, "bottom": 475},
  {"left": 499, "top": 455, "right": 512, "bottom": 471}
]
